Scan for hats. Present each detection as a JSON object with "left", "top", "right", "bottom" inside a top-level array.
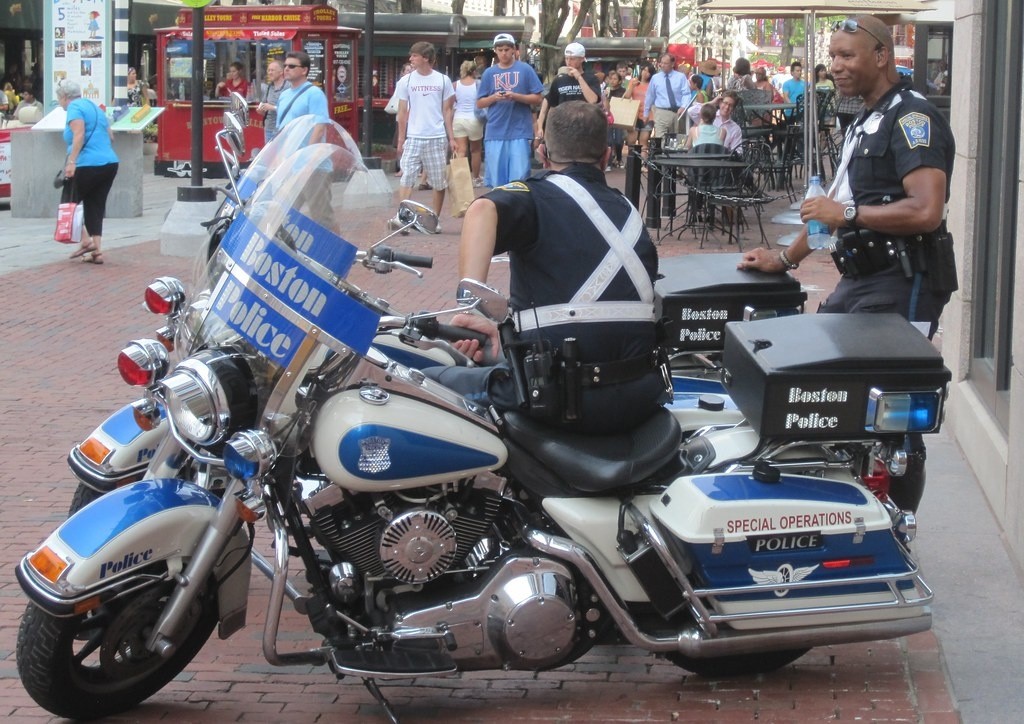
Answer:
[
  {"left": 565, "top": 43, "right": 587, "bottom": 63},
  {"left": 733, "top": 58, "right": 750, "bottom": 74},
  {"left": 493, "top": 33, "right": 515, "bottom": 53},
  {"left": 779, "top": 67, "right": 786, "bottom": 72},
  {"left": 698, "top": 60, "right": 721, "bottom": 75}
]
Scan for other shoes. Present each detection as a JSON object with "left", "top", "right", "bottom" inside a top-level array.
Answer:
[
  {"left": 395, "top": 170, "right": 403, "bottom": 177},
  {"left": 418, "top": 182, "right": 433, "bottom": 190},
  {"left": 603, "top": 158, "right": 648, "bottom": 172}
]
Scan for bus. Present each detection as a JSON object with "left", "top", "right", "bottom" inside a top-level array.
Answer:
[{"left": 153, "top": 1, "right": 534, "bottom": 180}]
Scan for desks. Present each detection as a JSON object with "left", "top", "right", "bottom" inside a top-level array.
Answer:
[
  {"left": 735, "top": 101, "right": 820, "bottom": 179},
  {"left": 648, "top": 159, "right": 754, "bottom": 241},
  {"left": 654, "top": 152, "right": 736, "bottom": 222}
]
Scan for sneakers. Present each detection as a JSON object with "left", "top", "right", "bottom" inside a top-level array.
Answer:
[
  {"left": 473, "top": 175, "right": 484, "bottom": 187},
  {"left": 415, "top": 223, "right": 441, "bottom": 234},
  {"left": 387, "top": 212, "right": 410, "bottom": 234}
]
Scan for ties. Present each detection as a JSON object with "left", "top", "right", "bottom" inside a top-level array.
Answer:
[{"left": 665, "top": 75, "right": 678, "bottom": 113}]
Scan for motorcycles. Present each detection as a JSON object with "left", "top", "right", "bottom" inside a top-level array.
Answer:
[
  {"left": 67, "top": 91, "right": 809, "bottom": 513},
  {"left": 12, "top": 144, "right": 939, "bottom": 723}
]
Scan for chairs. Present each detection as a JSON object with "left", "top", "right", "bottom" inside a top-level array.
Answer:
[{"left": 639, "top": 85, "right": 842, "bottom": 252}]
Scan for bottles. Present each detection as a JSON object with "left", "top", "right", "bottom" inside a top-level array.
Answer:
[
  {"left": 805, "top": 177, "right": 831, "bottom": 249},
  {"left": 674, "top": 139, "right": 677, "bottom": 148},
  {"left": 179, "top": 83, "right": 185, "bottom": 100},
  {"left": 669, "top": 139, "right": 674, "bottom": 148}
]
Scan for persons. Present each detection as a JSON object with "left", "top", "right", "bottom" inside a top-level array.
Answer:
[
  {"left": 55, "top": 81, "right": 119, "bottom": 264},
  {"left": 127, "top": 67, "right": 150, "bottom": 106},
  {"left": 453, "top": 101, "right": 671, "bottom": 436},
  {"left": 275, "top": 52, "right": 340, "bottom": 239},
  {"left": 735, "top": 12, "right": 959, "bottom": 343},
  {"left": 387, "top": 42, "right": 455, "bottom": 236},
  {"left": 392, "top": 54, "right": 950, "bottom": 225},
  {"left": 215, "top": 62, "right": 250, "bottom": 99},
  {"left": 475, "top": 32, "right": 544, "bottom": 187},
  {"left": 255, "top": 60, "right": 291, "bottom": 144},
  {"left": 536, "top": 42, "right": 602, "bottom": 139},
  {"left": 0, "top": 62, "right": 43, "bottom": 123}
]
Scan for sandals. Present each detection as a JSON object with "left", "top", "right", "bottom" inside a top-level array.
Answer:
[
  {"left": 70, "top": 241, "right": 97, "bottom": 258},
  {"left": 83, "top": 252, "right": 104, "bottom": 264}
]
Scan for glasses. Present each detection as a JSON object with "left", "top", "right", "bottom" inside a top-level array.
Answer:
[
  {"left": 830, "top": 20, "right": 885, "bottom": 47},
  {"left": 721, "top": 101, "right": 733, "bottom": 107},
  {"left": 283, "top": 64, "right": 308, "bottom": 69}
]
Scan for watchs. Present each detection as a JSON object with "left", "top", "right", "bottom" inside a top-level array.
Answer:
[{"left": 842, "top": 205, "right": 859, "bottom": 230}]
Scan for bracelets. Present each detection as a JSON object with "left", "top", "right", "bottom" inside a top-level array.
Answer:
[
  {"left": 779, "top": 249, "right": 800, "bottom": 270},
  {"left": 67, "top": 157, "right": 77, "bottom": 164}
]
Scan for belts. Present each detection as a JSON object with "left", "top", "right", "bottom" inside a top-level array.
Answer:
[
  {"left": 574, "top": 352, "right": 650, "bottom": 387},
  {"left": 656, "top": 107, "right": 680, "bottom": 110}
]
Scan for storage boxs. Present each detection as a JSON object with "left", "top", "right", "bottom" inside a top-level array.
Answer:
[
  {"left": 652, "top": 254, "right": 808, "bottom": 352},
  {"left": 720, "top": 312, "right": 951, "bottom": 443}
]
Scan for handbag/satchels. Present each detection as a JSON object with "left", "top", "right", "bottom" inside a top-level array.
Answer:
[
  {"left": 449, "top": 151, "right": 474, "bottom": 217},
  {"left": 609, "top": 90, "right": 641, "bottom": 130},
  {"left": 474, "top": 80, "right": 488, "bottom": 123},
  {"left": 53, "top": 168, "right": 71, "bottom": 188},
  {"left": 54, "top": 182, "right": 83, "bottom": 243},
  {"left": 139, "top": 80, "right": 151, "bottom": 107}
]
